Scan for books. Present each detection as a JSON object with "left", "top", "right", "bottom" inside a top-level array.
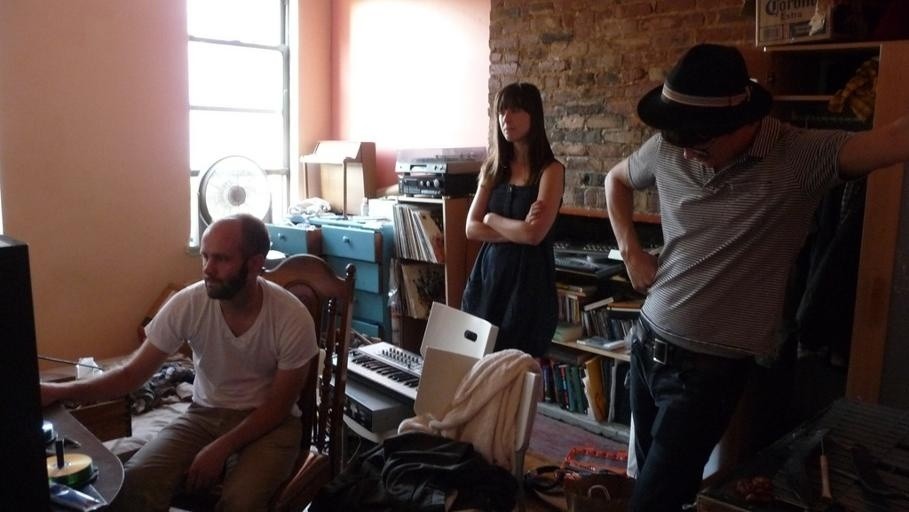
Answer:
[
  {"left": 392, "top": 202, "right": 444, "bottom": 320},
  {"left": 537, "top": 280, "right": 645, "bottom": 430}
]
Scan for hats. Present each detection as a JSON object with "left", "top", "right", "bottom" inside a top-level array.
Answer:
[{"left": 637, "top": 42, "right": 773, "bottom": 148}]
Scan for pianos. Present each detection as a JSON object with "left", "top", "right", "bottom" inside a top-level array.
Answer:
[{"left": 334, "top": 340, "right": 423, "bottom": 402}]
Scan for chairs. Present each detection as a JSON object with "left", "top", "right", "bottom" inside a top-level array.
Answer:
[
  {"left": 245, "top": 253, "right": 361, "bottom": 512},
  {"left": 402, "top": 296, "right": 542, "bottom": 511}
]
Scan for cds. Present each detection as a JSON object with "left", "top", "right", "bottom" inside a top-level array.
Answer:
[{"left": 46, "top": 452, "right": 94, "bottom": 487}]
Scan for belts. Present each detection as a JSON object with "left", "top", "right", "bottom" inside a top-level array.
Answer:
[{"left": 636, "top": 320, "right": 694, "bottom": 364}]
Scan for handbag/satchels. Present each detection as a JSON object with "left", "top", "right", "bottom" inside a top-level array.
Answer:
[{"left": 562, "top": 472, "right": 636, "bottom": 512}]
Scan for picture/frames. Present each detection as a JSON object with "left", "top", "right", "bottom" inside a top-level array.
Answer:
[{"left": 755, "top": 0, "right": 833, "bottom": 49}]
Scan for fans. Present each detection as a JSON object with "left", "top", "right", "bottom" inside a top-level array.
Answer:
[{"left": 196, "top": 153, "right": 274, "bottom": 233}]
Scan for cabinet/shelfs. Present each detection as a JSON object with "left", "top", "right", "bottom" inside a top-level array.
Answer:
[
  {"left": 392, "top": 196, "right": 472, "bottom": 349},
  {"left": 759, "top": 37, "right": 907, "bottom": 411},
  {"left": 262, "top": 213, "right": 396, "bottom": 350},
  {"left": 539, "top": 206, "right": 664, "bottom": 444}
]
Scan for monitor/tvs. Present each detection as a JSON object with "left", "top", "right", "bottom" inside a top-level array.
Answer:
[{"left": 0, "top": 234, "right": 52, "bottom": 512}]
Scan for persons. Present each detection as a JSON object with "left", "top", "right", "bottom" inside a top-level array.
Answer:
[
  {"left": 460, "top": 82, "right": 567, "bottom": 358},
  {"left": 601, "top": 42, "right": 907, "bottom": 512},
  {"left": 39, "top": 214, "right": 320, "bottom": 512}
]
